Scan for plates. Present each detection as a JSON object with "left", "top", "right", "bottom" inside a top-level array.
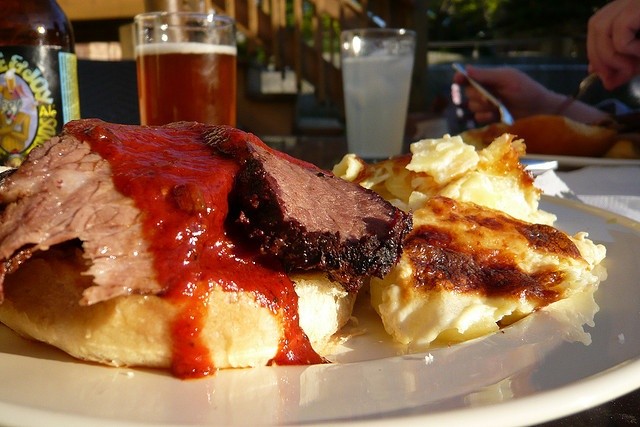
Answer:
[{"left": 0, "top": 194, "right": 640, "bottom": 427}]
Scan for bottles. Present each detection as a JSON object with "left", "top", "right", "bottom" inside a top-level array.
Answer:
[{"left": 0, "top": 0, "right": 82, "bottom": 168}]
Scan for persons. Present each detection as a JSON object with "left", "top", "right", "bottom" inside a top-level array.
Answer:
[{"left": 447, "top": 1, "right": 636, "bottom": 123}]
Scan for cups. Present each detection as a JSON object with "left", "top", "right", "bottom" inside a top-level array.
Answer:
[
  {"left": 339, "top": 27, "right": 418, "bottom": 160},
  {"left": 133, "top": 11, "right": 238, "bottom": 125}
]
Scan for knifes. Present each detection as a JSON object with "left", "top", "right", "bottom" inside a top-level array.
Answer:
[{"left": 554, "top": 67, "right": 602, "bottom": 117}]
